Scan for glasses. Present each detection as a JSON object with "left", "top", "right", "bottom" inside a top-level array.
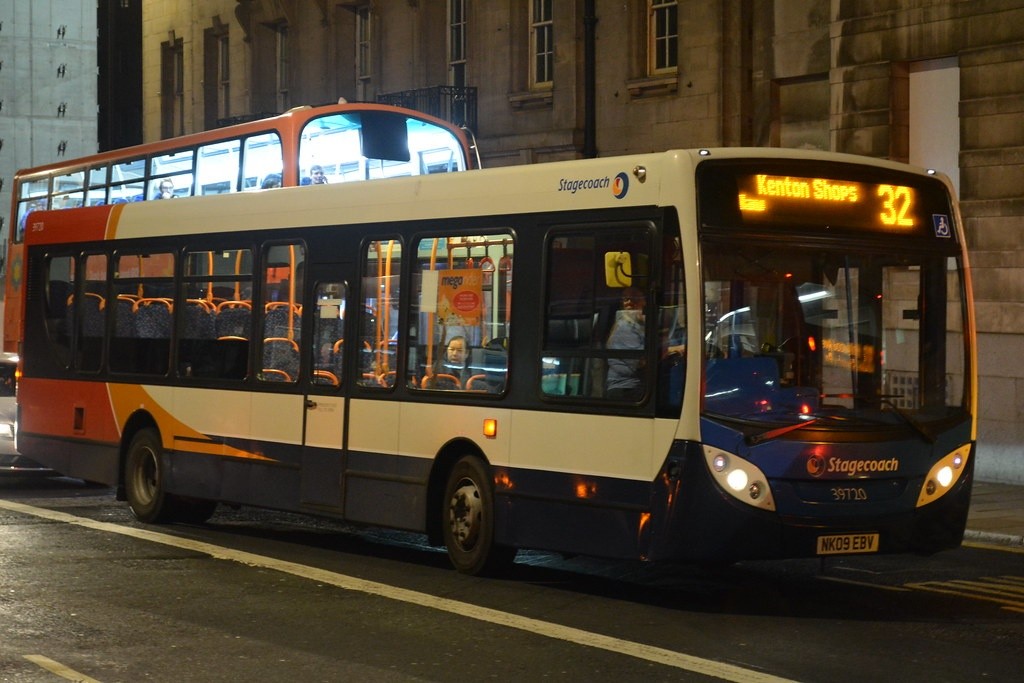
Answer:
[{"left": 621, "top": 294, "right": 640, "bottom": 303}]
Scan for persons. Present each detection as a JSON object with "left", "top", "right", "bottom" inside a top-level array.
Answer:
[
  {"left": 309, "top": 165, "right": 325, "bottom": 185},
  {"left": 602, "top": 283, "right": 687, "bottom": 403},
  {"left": 433, "top": 336, "right": 474, "bottom": 392},
  {"left": 263, "top": 175, "right": 282, "bottom": 189},
  {"left": 158, "top": 178, "right": 179, "bottom": 200}
]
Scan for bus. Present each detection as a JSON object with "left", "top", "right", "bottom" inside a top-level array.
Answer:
[
  {"left": 1, "top": 98, "right": 484, "bottom": 370},
  {"left": 12, "top": 147, "right": 979, "bottom": 575}
]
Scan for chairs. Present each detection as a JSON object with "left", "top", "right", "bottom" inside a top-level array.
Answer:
[
  {"left": 65, "top": 292, "right": 103, "bottom": 336},
  {"left": 342, "top": 305, "right": 384, "bottom": 350},
  {"left": 184, "top": 299, "right": 215, "bottom": 339},
  {"left": 132, "top": 298, "right": 171, "bottom": 339},
  {"left": 378, "top": 371, "right": 416, "bottom": 388},
  {"left": 262, "top": 368, "right": 291, "bottom": 382},
  {"left": 421, "top": 374, "right": 462, "bottom": 390},
  {"left": 314, "top": 369, "right": 339, "bottom": 386},
  {"left": 363, "top": 374, "right": 388, "bottom": 388},
  {"left": 264, "top": 337, "right": 299, "bottom": 381},
  {"left": 466, "top": 375, "right": 494, "bottom": 392},
  {"left": 589, "top": 304, "right": 617, "bottom": 398},
  {"left": 98, "top": 296, "right": 136, "bottom": 337},
  {"left": 215, "top": 301, "right": 252, "bottom": 338},
  {"left": 333, "top": 339, "right": 373, "bottom": 380},
  {"left": 264, "top": 302, "right": 301, "bottom": 341}
]
